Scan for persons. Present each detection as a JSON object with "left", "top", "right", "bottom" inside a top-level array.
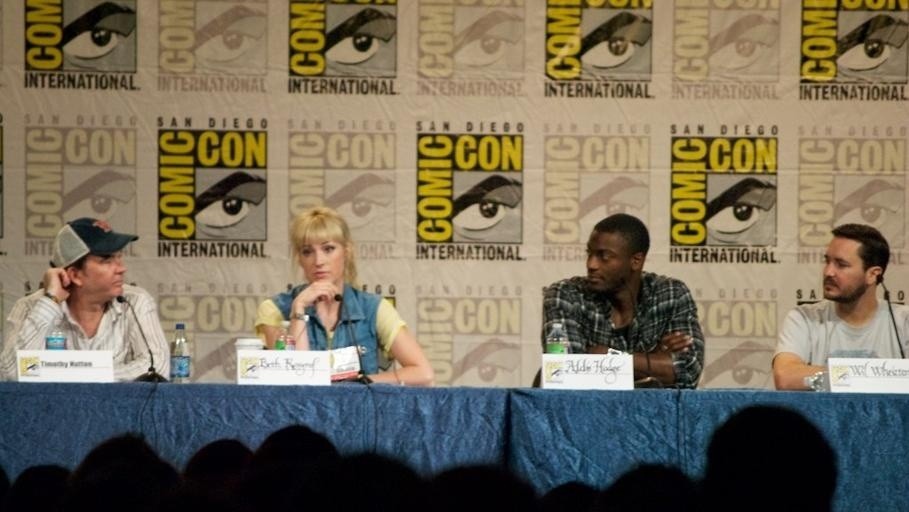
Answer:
[
  {"left": 256, "top": 207, "right": 436, "bottom": 385},
  {"left": 541, "top": 403, "right": 836, "bottom": 511},
  {"left": 542, "top": 214, "right": 705, "bottom": 389},
  {"left": 0, "top": 217, "right": 170, "bottom": 382},
  {"left": 1, "top": 423, "right": 541, "bottom": 511},
  {"left": 771, "top": 226, "right": 909, "bottom": 390}
]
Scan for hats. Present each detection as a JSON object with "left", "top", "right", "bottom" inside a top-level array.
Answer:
[{"left": 50, "top": 217, "right": 139, "bottom": 268}]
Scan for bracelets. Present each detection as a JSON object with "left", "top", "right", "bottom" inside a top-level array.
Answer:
[
  {"left": 393, "top": 368, "right": 404, "bottom": 386},
  {"left": 43, "top": 293, "right": 63, "bottom": 305}
]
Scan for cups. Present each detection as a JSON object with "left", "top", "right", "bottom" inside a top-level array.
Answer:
[{"left": 235, "top": 338, "right": 264, "bottom": 350}]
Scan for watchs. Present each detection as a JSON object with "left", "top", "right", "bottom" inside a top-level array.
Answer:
[{"left": 288, "top": 312, "right": 310, "bottom": 323}]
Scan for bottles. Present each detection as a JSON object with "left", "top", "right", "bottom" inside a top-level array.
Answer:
[
  {"left": 45, "top": 318, "right": 68, "bottom": 347},
  {"left": 803, "top": 371, "right": 830, "bottom": 392},
  {"left": 274, "top": 320, "right": 295, "bottom": 349},
  {"left": 547, "top": 324, "right": 569, "bottom": 355},
  {"left": 171, "top": 323, "right": 191, "bottom": 383}
]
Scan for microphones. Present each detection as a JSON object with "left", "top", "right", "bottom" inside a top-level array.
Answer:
[
  {"left": 332, "top": 290, "right": 377, "bottom": 387},
  {"left": 611, "top": 275, "right": 670, "bottom": 386},
  {"left": 874, "top": 272, "right": 909, "bottom": 358},
  {"left": 115, "top": 293, "right": 170, "bottom": 384}
]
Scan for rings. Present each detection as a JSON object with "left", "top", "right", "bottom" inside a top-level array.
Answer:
[{"left": 660, "top": 345, "right": 669, "bottom": 352}]
